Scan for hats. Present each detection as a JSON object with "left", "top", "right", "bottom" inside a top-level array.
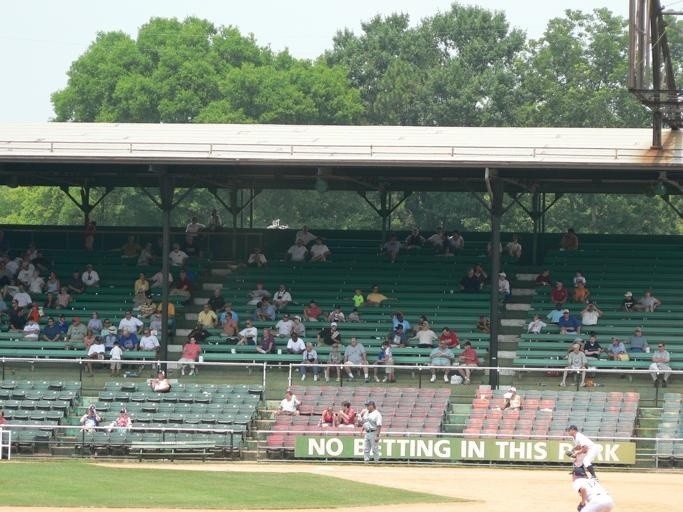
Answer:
[
  {"left": 570, "top": 468, "right": 584, "bottom": 474},
  {"left": 285, "top": 314, "right": 289, "bottom": 318},
  {"left": 120, "top": 408, "right": 126, "bottom": 413},
  {"left": 331, "top": 322, "right": 337, "bottom": 327},
  {"left": 365, "top": 401, "right": 375, "bottom": 405},
  {"left": 566, "top": 425, "right": 577, "bottom": 430},
  {"left": 157, "top": 370, "right": 164, "bottom": 376},
  {"left": 395, "top": 324, "right": 403, "bottom": 329},
  {"left": 89, "top": 405, "right": 95, "bottom": 410}
]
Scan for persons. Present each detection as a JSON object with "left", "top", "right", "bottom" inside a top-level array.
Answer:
[{"left": 568, "top": 468, "right": 614, "bottom": 512}]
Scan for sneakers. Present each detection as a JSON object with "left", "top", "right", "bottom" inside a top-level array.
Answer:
[
  {"left": 431, "top": 374, "right": 436, "bottom": 383},
  {"left": 662, "top": 380, "right": 667, "bottom": 387},
  {"left": 347, "top": 376, "right": 356, "bottom": 382},
  {"left": 373, "top": 376, "right": 380, "bottom": 382},
  {"left": 580, "top": 382, "right": 585, "bottom": 387},
  {"left": 314, "top": 374, "right": 318, "bottom": 381},
  {"left": 559, "top": 382, "right": 567, "bottom": 386},
  {"left": 464, "top": 376, "right": 471, "bottom": 385},
  {"left": 444, "top": 374, "right": 449, "bottom": 382},
  {"left": 655, "top": 380, "right": 660, "bottom": 388},
  {"left": 301, "top": 374, "right": 306, "bottom": 381},
  {"left": 383, "top": 376, "right": 387, "bottom": 382},
  {"left": 364, "top": 378, "right": 369, "bottom": 383}
]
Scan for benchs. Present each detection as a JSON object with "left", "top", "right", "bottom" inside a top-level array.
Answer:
[{"left": 0, "top": 219, "right": 682, "bottom": 473}]
